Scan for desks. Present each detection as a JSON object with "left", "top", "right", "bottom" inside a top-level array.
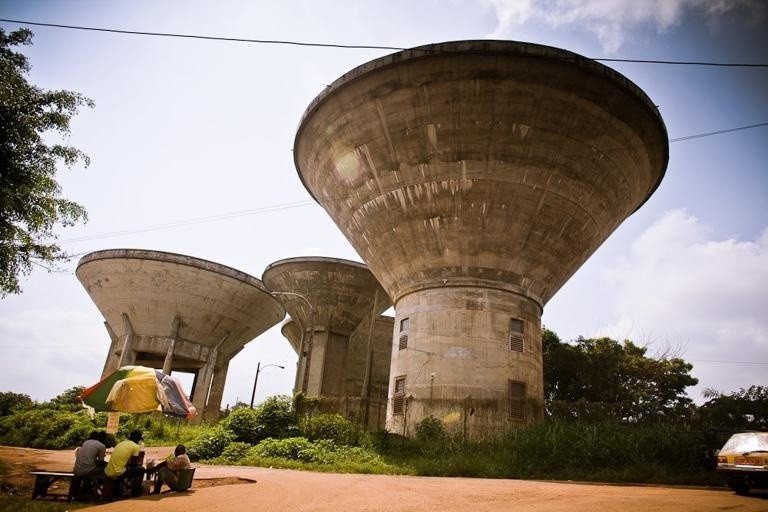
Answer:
[{"left": 28, "top": 471, "right": 77, "bottom": 502}]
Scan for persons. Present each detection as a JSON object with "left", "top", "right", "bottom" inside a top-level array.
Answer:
[
  {"left": 73, "top": 429, "right": 107, "bottom": 498},
  {"left": 148, "top": 445, "right": 191, "bottom": 495},
  {"left": 104, "top": 430, "right": 146, "bottom": 498}
]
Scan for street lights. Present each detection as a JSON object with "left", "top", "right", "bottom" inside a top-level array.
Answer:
[
  {"left": 269, "top": 291, "right": 312, "bottom": 396},
  {"left": 249, "top": 361, "right": 286, "bottom": 409}
]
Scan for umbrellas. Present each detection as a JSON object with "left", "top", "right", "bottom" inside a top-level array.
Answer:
[{"left": 76, "top": 364, "right": 199, "bottom": 441}]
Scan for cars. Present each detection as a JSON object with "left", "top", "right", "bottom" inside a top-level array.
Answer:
[{"left": 711, "top": 429, "right": 767, "bottom": 494}]
